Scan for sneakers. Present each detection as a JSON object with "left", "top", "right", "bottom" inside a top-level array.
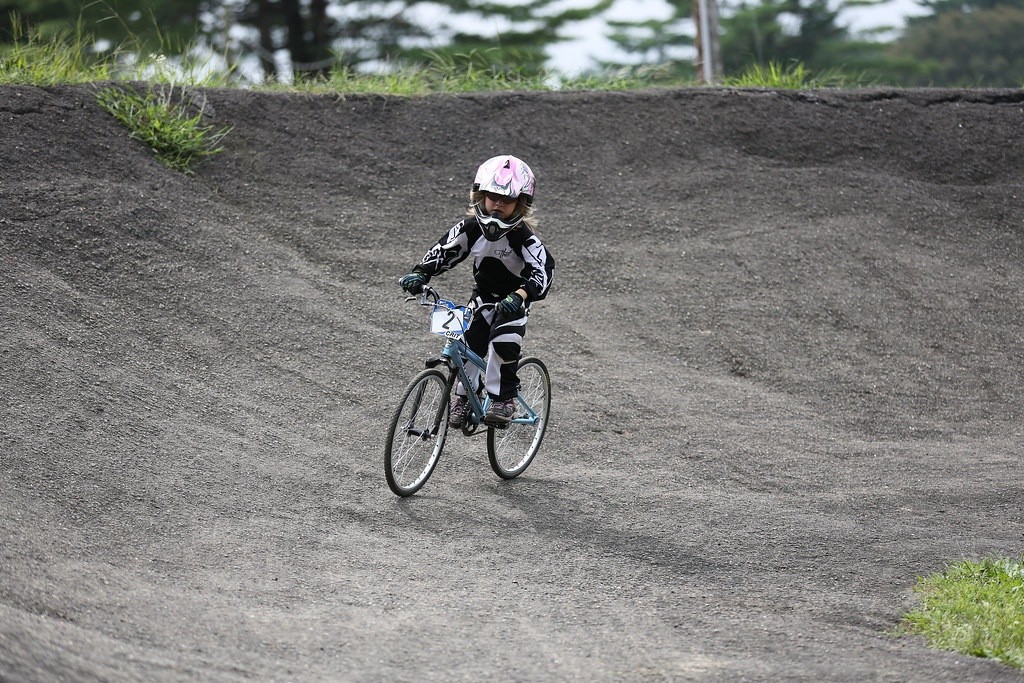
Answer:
[
  {"left": 486, "top": 397, "right": 517, "bottom": 422},
  {"left": 447, "top": 393, "right": 468, "bottom": 427}
]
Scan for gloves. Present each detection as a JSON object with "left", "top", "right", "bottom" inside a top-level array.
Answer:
[
  {"left": 498, "top": 292, "right": 523, "bottom": 319},
  {"left": 400, "top": 273, "right": 426, "bottom": 297}
]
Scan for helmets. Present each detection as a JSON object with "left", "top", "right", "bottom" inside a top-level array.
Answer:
[{"left": 470, "top": 155, "right": 537, "bottom": 242}]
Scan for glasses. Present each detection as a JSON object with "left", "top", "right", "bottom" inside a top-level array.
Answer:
[{"left": 483, "top": 189, "right": 518, "bottom": 204}]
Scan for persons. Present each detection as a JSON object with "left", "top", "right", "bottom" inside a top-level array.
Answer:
[{"left": 399, "top": 156, "right": 556, "bottom": 428}]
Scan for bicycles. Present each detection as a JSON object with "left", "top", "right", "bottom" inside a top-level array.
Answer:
[{"left": 384, "top": 277, "right": 552, "bottom": 498}]
